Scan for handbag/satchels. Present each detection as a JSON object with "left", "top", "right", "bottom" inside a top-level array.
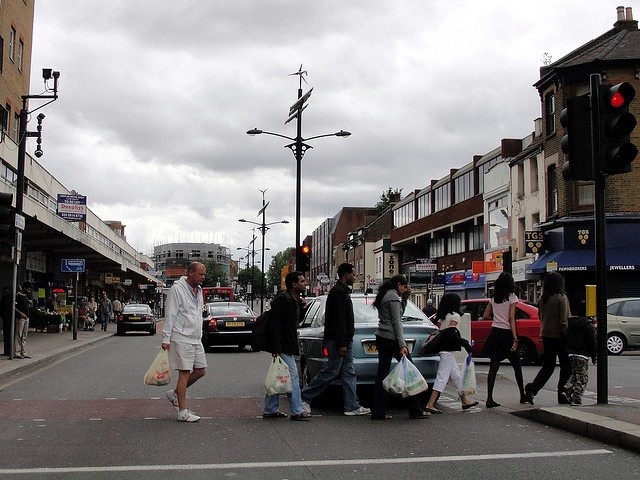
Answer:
[
  {"left": 94, "top": 312, "right": 98, "bottom": 320},
  {"left": 251, "top": 309, "right": 271, "bottom": 352},
  {"left": 80, "top": 309, "right": 87, "bottom": 315}
]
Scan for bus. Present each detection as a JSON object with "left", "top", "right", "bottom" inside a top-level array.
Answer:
[{"left": 202, "top": 287, "right": 234, "bottom": 305}]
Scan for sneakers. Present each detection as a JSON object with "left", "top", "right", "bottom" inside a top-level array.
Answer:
[
  {"left": 290, "top": 412, "right": 312, "bottom": 420},
  {"left": 166, "top": 390, "right": 180, "bottom": 413},
  {"left": 176, "top": 412, "right": 201, "bottom": 422},
  {"left": 520, "top": 397, "right": 534, "bottom": 403},
  {"left": 300, "top": 397, "right": 312, "bottom": 414},
  {"left": 263, "top": 412, "right": 288, "bottom": 418},
  {"left": 344, "top": 406, "right": 371, "bottom": 416},
  {"left": 104, "top": 328, "right": 107, "bottom": 331},
  {"left": 486, "top": 400, "right": 500, "bottom": 407},
  {"left": 561, "top": 391, "right": 571, "bottom": 403},
  {"left": 570, "top": 401, "right": 582, "bottom": 406},
  {"left": 22, "top": 352, "right": 28, "bottom": 358},
  {"left": 101, "top": 327, "right": 103, "bottom": 330},
  {"left": 15, "top": 353, "right": 21, "bottom": 358},
  {"left": 525, "top": 386, "right": 534, "bottom": 405}
]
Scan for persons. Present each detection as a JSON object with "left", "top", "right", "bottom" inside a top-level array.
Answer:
[
  {"left": 422, "top": 299, "right": 436, "bottom": 318},
  {"left": 524, "top": 271, "right": 574, "bottom": 402},
  {"left": 370, "top": 275, "right": 431, "bottom": 419},
  {"left": 482, "top": 272, "right": 534, "bottom": 408},
  {"left": 299, "top": 264, "right": 372, "bottom": 416},
  {"left": 423, "top": 293, "right": 479, "bottom": 415},
  {"left": 122, "top": 298, "right": 143, "bottom": 312},
  {"left": 559, "top": 300, "right": 597, "bottom": 405},
  {"left": 88, "top": 296, "right": 97, "bottom": 320},
  {"left": 101, "top": 293, "right": 113, "bottom": 331},
  {"left": 112, "top": 297, "right": 121, "bottom": 325},
  {"left": 14, "top": 282, "right": 34, "bottom": 359},
  {"left": 161, "top": 260, "right": 208, "bottom": 423},
  {"left": 261, "top": 271, "right": 314, "bottom": 419},
  {"left": 79, "top": 300, "right": 93, "bottom": 330}
]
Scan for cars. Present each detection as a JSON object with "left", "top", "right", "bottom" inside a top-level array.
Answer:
[
  {"left": 117, "top": 303, "right": 155, "bottom": 336},
  {"left": 592, "top": 296, "right": 640, "bottom": 354},
  {"left": 294, "top": 294, "right": 449, "bottom": 407},
  {"left": 201, "top": 302, "right": 260, "bottom": 351},
  {"left": 429, "top": 298, "right": 544, "bottom": 362}
]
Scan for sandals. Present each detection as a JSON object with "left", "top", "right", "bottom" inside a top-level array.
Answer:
[
  {"left": 420, "top": 410, "right": 431, "bottom": 416},
  {"left": 371, "top": 415, "right": 393, "bottom": 420}
]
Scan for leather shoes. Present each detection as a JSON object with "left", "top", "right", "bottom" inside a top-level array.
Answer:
[
  {"left": 425, "top": 407, "right": 443, "bottom": 414},
  {"left": 462, "top": 401, "right": 479, "bottom": 409}
]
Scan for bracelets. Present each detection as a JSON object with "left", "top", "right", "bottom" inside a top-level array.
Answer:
[{"left": 513, "top": 338, "right": 520, "bottom": 343}]
